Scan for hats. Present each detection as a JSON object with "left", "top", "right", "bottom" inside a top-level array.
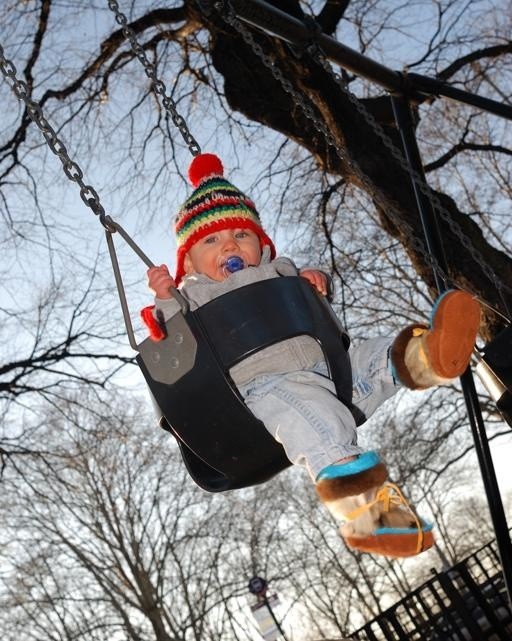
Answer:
[{"left": 175, "top": 154, "right": 276, "bottom": 284}]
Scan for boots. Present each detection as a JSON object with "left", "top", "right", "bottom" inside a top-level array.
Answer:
[
  {"left": 391, "top": 290, "right": 480, "bottom": 389},
  {"left": 316, "top": 452, "right": 433, "bottom": 556}
]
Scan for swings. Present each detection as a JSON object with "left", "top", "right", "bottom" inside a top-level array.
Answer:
[{"left": 1, "top": 0, "right": 367, "bottom": 493}]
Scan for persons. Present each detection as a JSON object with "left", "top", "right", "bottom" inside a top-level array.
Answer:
[{"left": 144, "top": 152, "right": 483, "bottom": 559}]
